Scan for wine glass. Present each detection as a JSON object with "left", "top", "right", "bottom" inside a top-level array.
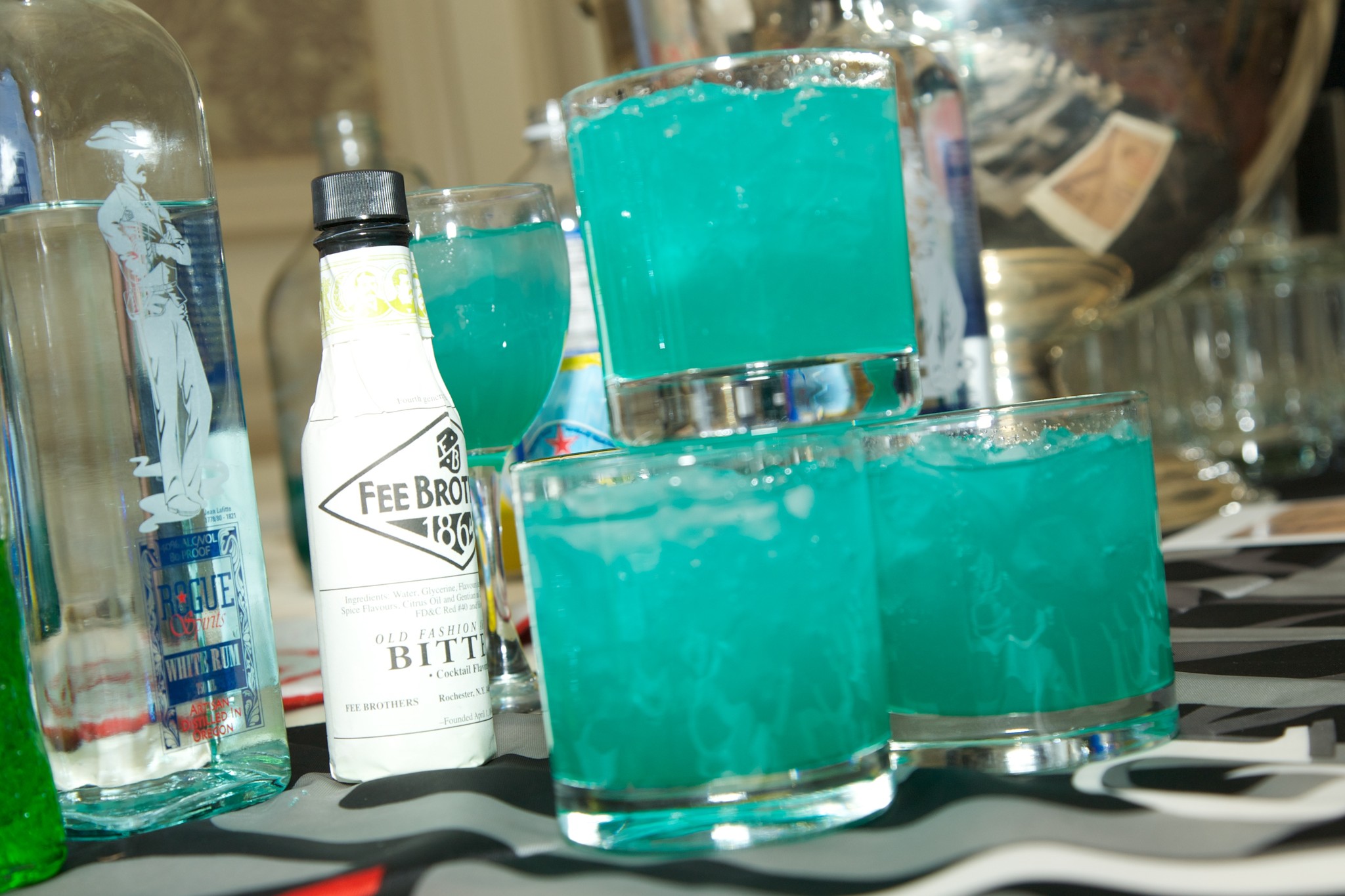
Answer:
[{"left": 403, "top": 181, "right": 572, "bottom": 718}]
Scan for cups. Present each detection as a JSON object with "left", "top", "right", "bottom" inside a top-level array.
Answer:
[
  {"left": 857, "top": 392, "right": 1179, "bottom": 772},
  {"left": 507, "top": 422, "right": 894, "bottom": 856},
  {"left": 559, "top": 46, "right": 925, "bottom": 441}
]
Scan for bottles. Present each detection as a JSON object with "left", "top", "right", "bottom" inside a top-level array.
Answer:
[
  {"left": 482, "top": 98, "right": 616, "bottom": 462},
  {"left": 267, "top": 103, "right": 440, "bottom": 577},
  {"left": 0, "top": 536, "right": 70, "bottom": 896},
  {"left": 1, "top": 0, "right": 294, "bottom": 842},
  {"left": 299, "top": 167, "right": 498, "bottom": 785},
  {"left": 772, "top": 1, "right": 994, "bottom": 434},
  {"left": 980, "top": 217, "right": 1344, "bottom": 545}
]
